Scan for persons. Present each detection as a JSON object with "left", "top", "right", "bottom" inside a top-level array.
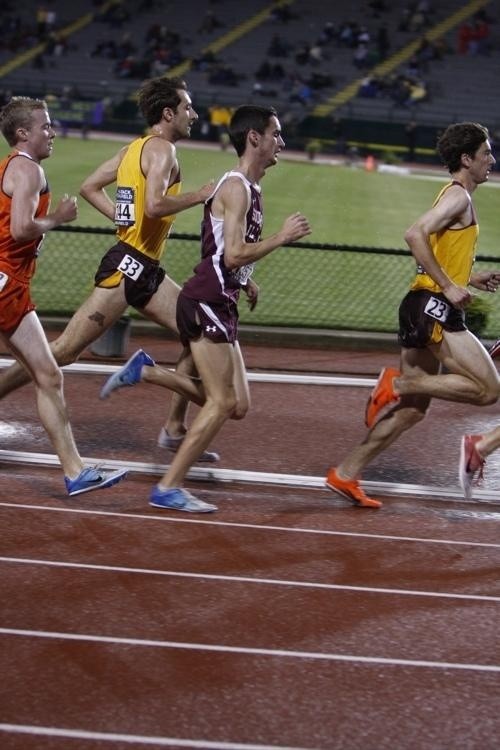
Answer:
[
  {"left": 323, "top": 120, "right": 499, "bottom": 509},
  {"left": 2, "top": 1, "right": 500, "bottom": 166},
  {"left": 0, "top": 77, "right": 223, "bottom": 463},
  {"left": 98, "top": 103, "right": 311, "bottom": 513},
  {"left": 458, "top": 414, "right": 499, "bottom": 497},
  {"left": 1, "top": 96, "right": 131, "bottom": 500}
]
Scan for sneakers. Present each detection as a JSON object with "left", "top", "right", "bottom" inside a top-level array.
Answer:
[
  {"left": 156, "top": 427, "right": 219, "bottom": 462},
  {"left": 459, "top": 434, "right": 485, "bottom": 497},
  {"left": 325, "top": 468, "right": 383, "bottom": 509},
  {"left": 365, "top": 367, "right": 401, "bottom": 429},
  {"left": 100, "top": 349, "right": 155, "bottom": 399},
  {"left": 148, "top": 486, "right": 218, "bottom": 513},
  {"left": 65, "top": 466, "right": 128, "bottom": 496}
]
